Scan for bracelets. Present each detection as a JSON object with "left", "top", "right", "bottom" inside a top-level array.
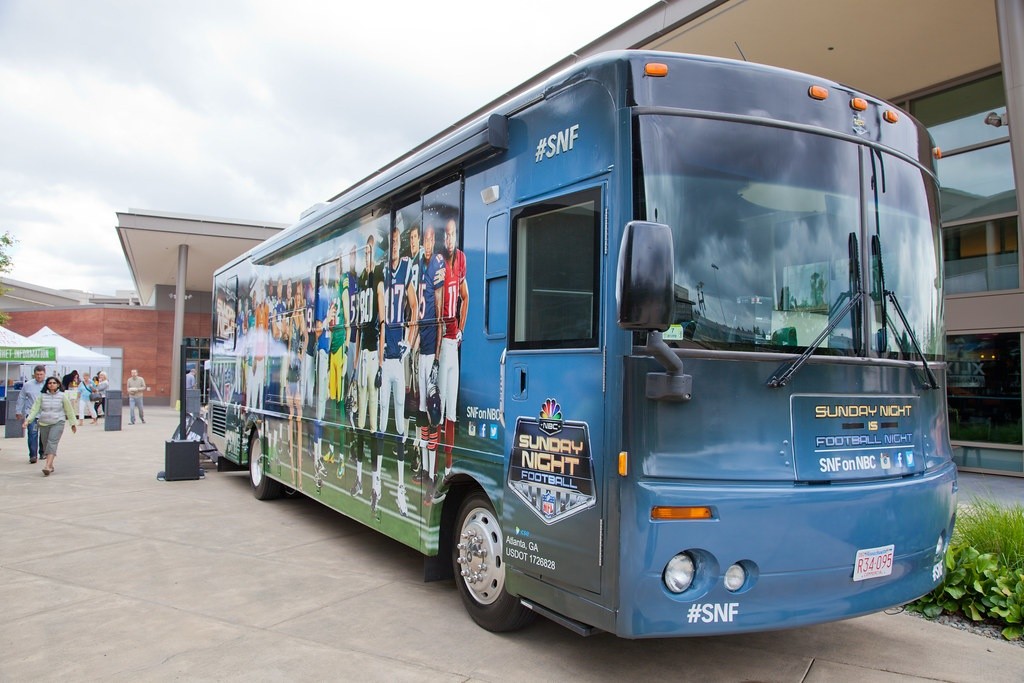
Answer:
[{"left": 459, "top": 328, "right": 464, "bottom": 336}]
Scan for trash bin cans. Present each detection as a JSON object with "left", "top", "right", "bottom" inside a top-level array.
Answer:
[
  {"left": 104, "top": 390, "right": 122, "bottom": 431},
  {"left": 186, "top": 389, "right": 200, "bottom": 416},
  {"left": 5, "top": 390, "right": 25, "bottom": 438}
]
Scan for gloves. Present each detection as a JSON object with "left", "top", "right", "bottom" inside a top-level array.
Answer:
[
  {"left": 349, "top": 369, "right": 356, "bottom": 388},
  {"left": 295, "top": 358, "right": 302, "bottom": 376},
  {"left": 426, "top": 360, "right": 439, "bottom": 384},
  {"left": 399, "top": 342, "right": 411, "bottom": 364},
  {"left": 342, "top": 345, "right": 349, "bottom": 364},
  {"left": 374, "top": 366, "right": 383, "bottom": 388},
  {"left": 455, "top": 329, "right": 464, "bottom": 346}
]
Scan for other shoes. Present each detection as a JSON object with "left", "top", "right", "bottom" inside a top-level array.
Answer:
[
  {"left": 77, "top": 422, "right": 84, "bottom": 426},
  {"left": 129, "top": 422, "right": 135, "bottom": 425},
  {"left": 92, "top": 421, "right": 98, "bottom": 426},
  {"left": 29, "top": 456, "right": 37, "bottom": 463},
  {"left": 431, "top": 471, "right": 453, "bottom": 505},
  {"left": 142, "top": 420, "right": 145, "bottom": 423},
  {"left": 39, "top": 454, "right": 47, "bottom": 459},
  {"left": 42, "top": 466, "right": 55, "bottom": 475}
]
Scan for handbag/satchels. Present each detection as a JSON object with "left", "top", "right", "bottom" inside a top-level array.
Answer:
[{"left": 89, "top": 388, "right": 103, "bottom": 401}]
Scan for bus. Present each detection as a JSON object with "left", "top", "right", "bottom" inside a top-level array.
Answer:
[{"left": 204, "top": 48, "right": 962, "bottom": 641}]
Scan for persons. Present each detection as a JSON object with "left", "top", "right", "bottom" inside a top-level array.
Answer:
[
  {"left": 93, "top": 371, "right": 109, "bottom": 419},
  {"left": 231, "top": 219, "right": 469, "bottom": 523},
  {"left": 61, "top": 370, "right": 81, "bottom": 390},
  {"left": 77, "top": 373, "right": 97, "bottom": 426},
  {"left": 186, "top": 369, "right": 197, "bottom": 390},
  {"left": 15, "top": 367, "right": 48, "bottom": 463},
  {"left": 21, "top": 377, "right": 77, "bottom": 476},
  {"left": 126, "top": 368, "right": 147, "bottom": 425}
]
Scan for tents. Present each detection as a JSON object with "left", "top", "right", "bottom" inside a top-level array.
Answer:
[
  {"left": 203, "top": 359, "right": 210, "bottom": 404},
  {"left": 5, "top": 326, "right": 111, "bottom": 418},
  {"left": 0, "top": 325, "right": 58, "bottom": 398}
]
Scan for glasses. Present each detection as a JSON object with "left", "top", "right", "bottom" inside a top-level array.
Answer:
[{"left": 47, "top": 382, "right": 57, "bottom": 384}]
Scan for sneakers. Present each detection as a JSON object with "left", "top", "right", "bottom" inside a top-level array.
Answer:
[
  {"left": 411, "top": 470, "right": 429, "bottom": 484},
  {"left": 374, "top": 476, "right": 382, "bottom": 500},
  {"left": 337, "top": 458, "right": 346, "bottom": 478},
  {"left": 317, "top": 458, "right": 328, "bottom": 477},
  {"left": 314, "top": 472, "right": 322, "bottom": 488},
  {"left": 350, "top": 475, "right": 364, "bottom": 497},
  {"left": 424, "top": 474, "right": 440, "bottom": 506},
  {"left": 396, "top": 484, "right": 409, "bottom": 516},
  {"left": 323, "top": 451, "right": 335, "bottom": 464},
  {"left": 369, "top": 489, "right": 378, "bottom": 516}
]
